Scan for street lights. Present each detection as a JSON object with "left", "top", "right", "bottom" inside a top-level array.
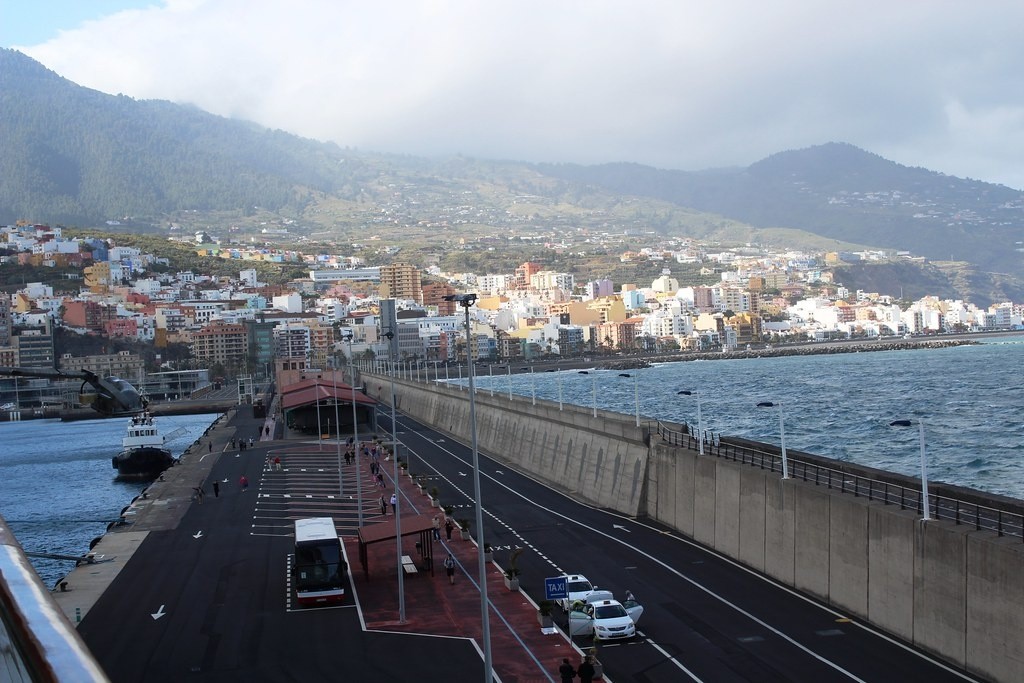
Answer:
[
  {"left": 442, "top": 292, "right": 496, "bottom": 683},
  {"left": 340, "top": 328, "right": 365, "bottom": 527},
  {"left": 379, "top": 330, "right": 407, "bottom": 622}
]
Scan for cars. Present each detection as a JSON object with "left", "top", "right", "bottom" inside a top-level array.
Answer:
[
  {"left": 557, "top": 573, "right": 600, "bottom": 612},
  {"left": 566, "top": 590, "right": 644, "bottom": 642}
]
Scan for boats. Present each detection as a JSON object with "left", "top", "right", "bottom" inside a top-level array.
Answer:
[{"left": 110, "top": 391, "right": 177, "bottom": 481}]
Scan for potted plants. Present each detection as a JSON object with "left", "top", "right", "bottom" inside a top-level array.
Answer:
[
  {"left": 444, "top": 506, "right": 453, "bottom": 521},
  {"left": 420, "top": 485, "right": 427, "bottom": 495},
  {"left": 503, "top": 547, "right": 519, "bottom": 590},
  {"left": 382, "top": 444, "right": 388, "bottom": 454},
  {"left": 388, "top": 448, "right": 394, "bottom": 461},
  {"left": 432, "top": 487, "right": 439, "bottom": 508},
  {"left": 377, "top": 440, "right": 383, "bottom": 448},
  {"left": 397, "top": 456, "right": 402, "bottom": 468},
  {"left": 372, "top": 435, "right": 378, "bottom": 443},
  {"left": 411, "top": 473, "right": 417, "bottom": 485},
  {"left": 582, "top": 643, "right": 603, "bottom": 679},
  {"left": 400, "top": 462, "right": 408, "bottom": 476},
  {"left": 537, "top": 600, "right": 553, "bottom": 629},
  {"left": 458, "top": 519, "right": 471, "bottom": 540},
  {"left": 484, "top": 542, "right": 494, "bottom": 562}
]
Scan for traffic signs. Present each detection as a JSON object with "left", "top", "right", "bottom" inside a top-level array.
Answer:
[{"left": 546, "top": 577, "right": 568, "bottom": 601}]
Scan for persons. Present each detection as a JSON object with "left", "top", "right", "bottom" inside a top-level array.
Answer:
[
  {"left": 577, "top": 656, "right": 595, "bottom": 683},
  {"left": 208, "top": 441, "right": 212, "bottom": 452},
  {"left": 444, "top": 554, "right": 455, "bottom": 584},
  {"left": 132, "top": 408, "right": 153, "bottom": 436},
  {"left": 559, "top": 658, "right": 576, "bottom": 683},
  {"left": 230, "top": 437, "right": 254, "bottom": 451},
  {"left": 344, "top": 436, "right": 386, "bottom": 488},
  {"left": 379, "top": 494, "right": 387, "bottom": 514},
  {"left": 259, "top": 425, "right": 270, "bottom": 436},
  {"left": 588, "top": 608, "right": 600, "bottom": 619},
  {"left": 240, "top": 474, "right": 247, "bottom": 492},
  {"left": 268, "top": 455, "right": 281, "bottom": 471},
  {"left": 432, "top": 515, "right": 441, "bottom": 542},
  {"left": 213, "top": 480, "right": 219, "bottom": 497},
  {"left": 625, "top": 590, "right": 635, "bottom": 603},
  {"left": 192, "top": 486, "right": 205, "bottom": 504},
  {"left": 444, "top": 517, "right": 453, "bottom": 541},
  {"left": 390, "top": 494, "right": 395, "bottom": 513}
]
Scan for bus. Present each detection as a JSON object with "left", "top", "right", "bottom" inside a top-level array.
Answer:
[{"left": 292, "top": 516, "right": 346, "bottom": 607}]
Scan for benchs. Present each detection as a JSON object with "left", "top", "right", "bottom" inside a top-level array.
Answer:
[{"left": 401, "top": 555, "right": 418, "bottom": 574}]
